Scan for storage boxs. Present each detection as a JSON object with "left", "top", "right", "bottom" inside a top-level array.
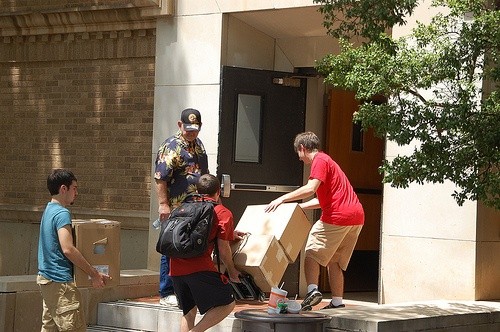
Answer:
[
  {"left": 72, "top": 218, "right": 121, "bottom": 287},
  {"left": 216, "top": 200, "right": 312, "bottom": 293}
]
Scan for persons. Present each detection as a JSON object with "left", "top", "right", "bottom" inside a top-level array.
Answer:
[
  {"left": 167, "top": 174, "right": 247, "bottom": 332},
  {"left": 263, "top": 131, "right": 365, "bottom": 311},
  {"left": 152, "top": 108, "right": 210, "bottom": 307},
  {"left": 35, "top": 169, "right": 109, "bottom": 332}
]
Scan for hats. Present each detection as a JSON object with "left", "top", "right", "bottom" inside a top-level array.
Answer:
[{"left": 181, "top": 109, "right": 202, "bottom": 131}]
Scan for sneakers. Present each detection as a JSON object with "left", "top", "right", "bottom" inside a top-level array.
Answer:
[
  {"left": 160, "top": 294, "right": 178, "bottom": 307},
  {"left": 301, "top": 288, "right": 323, "bottom": 311},
  {"left": 320, "top": 301, "right": 345, "bottom": 310}
]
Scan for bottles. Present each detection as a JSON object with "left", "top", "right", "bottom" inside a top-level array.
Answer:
[{"left": 152, "top": 218, "right": 161, "bottom": 230}]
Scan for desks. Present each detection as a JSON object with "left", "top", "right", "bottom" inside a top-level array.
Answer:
[{"left": 234, "top": 307, "right": 333, "bottom": 332}]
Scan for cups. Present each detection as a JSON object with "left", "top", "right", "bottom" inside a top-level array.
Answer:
[
  {"left": 286, "top": 299, "right": 302, "bottom": 313},
  {"left": 267, "top": 287, "right": 288, "bottom": 314}
]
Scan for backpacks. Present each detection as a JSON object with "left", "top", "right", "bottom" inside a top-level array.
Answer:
[{"left": 155, "top": 194, "right": 220, "bottom": 258}]
